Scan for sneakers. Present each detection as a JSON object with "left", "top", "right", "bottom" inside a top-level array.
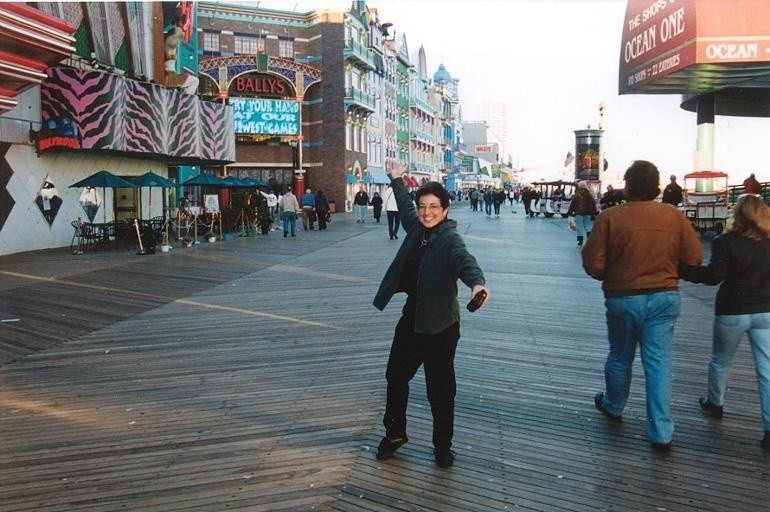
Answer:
[
  {"left": 595, "top": 394, "right": 622, "bottom": 422},
  {"left": 433, "top": 448, "right": 456, "bottom": 467},
  {"left": 377, "top": 436, "right": 408, "bottom": 460}
]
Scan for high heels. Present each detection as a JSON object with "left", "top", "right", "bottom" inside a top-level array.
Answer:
[{"left": 699, "top": 398, "right": 723, "bottom": 418}]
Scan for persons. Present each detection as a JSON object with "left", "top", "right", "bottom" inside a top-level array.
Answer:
[
  {"left": 567, "top": 180, "right": 596, "bottom": 247},
  {"left": 369, "top": 191, "right": 383, "bottom": 223},
  {"left": 373, "top": 158, "right": 490, "bottom": 468},
  {"left": 677, "top": 193, "right": 770, "bottom": 452},
  {"left": 382, "top": 185, "right": 401, "bottom": 240},
  {"left": 663, "top": 176, "right": 683, "bottom": 206},
  {"left": 259, "top": 186, "right": 331, "bottom": 238},
  {"left": 582, "top": 161, "right": 703, "bottom": 453},
  {"left": 743, "top": 174, "right": 761, "bottom": 196},
  {"left": 596, "top": 184, "right": 623, "bottom": 212},
  {"left": 447, "top": 185, "right": 565, "bottom": 218},
  {"left": 353, "top": 187, "right": 369, "bottom": 223}
]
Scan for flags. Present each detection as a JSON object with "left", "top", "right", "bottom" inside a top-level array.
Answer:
[{"left": 564, "top": 152, "right": 574, "bottom": 167}]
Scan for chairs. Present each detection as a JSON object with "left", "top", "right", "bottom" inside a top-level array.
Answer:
[{"left": 69, "top": 208, "right": 223, "bottom": 252}]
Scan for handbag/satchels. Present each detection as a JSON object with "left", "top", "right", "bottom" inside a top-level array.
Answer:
[
  {"left": 312, "top": 211, "right": 317, "bottom": 221},
  {"left": 325, "top": 212, "right": 331, "bottom": 221}
]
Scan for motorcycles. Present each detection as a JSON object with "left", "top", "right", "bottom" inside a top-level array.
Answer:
[{"left": 681, "top": 171, "right": 729, "bottom": 237}]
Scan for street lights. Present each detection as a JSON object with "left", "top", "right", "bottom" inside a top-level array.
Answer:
[{"left": 598, "top": 102, "right": 606, "bottom": 199}]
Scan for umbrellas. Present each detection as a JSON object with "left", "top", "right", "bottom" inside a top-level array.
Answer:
[
  {"left": 68, "top": 171, "right": 135, "bottom": 224},
  {"left": 222, "top": 168, "right": 272, "bottom": 224},
  {"left": 180, "top": 173, "right": 235, "bottom": 203},
  {"left": 129, "top": 170, "right": 177, "bottom": 220}
]
